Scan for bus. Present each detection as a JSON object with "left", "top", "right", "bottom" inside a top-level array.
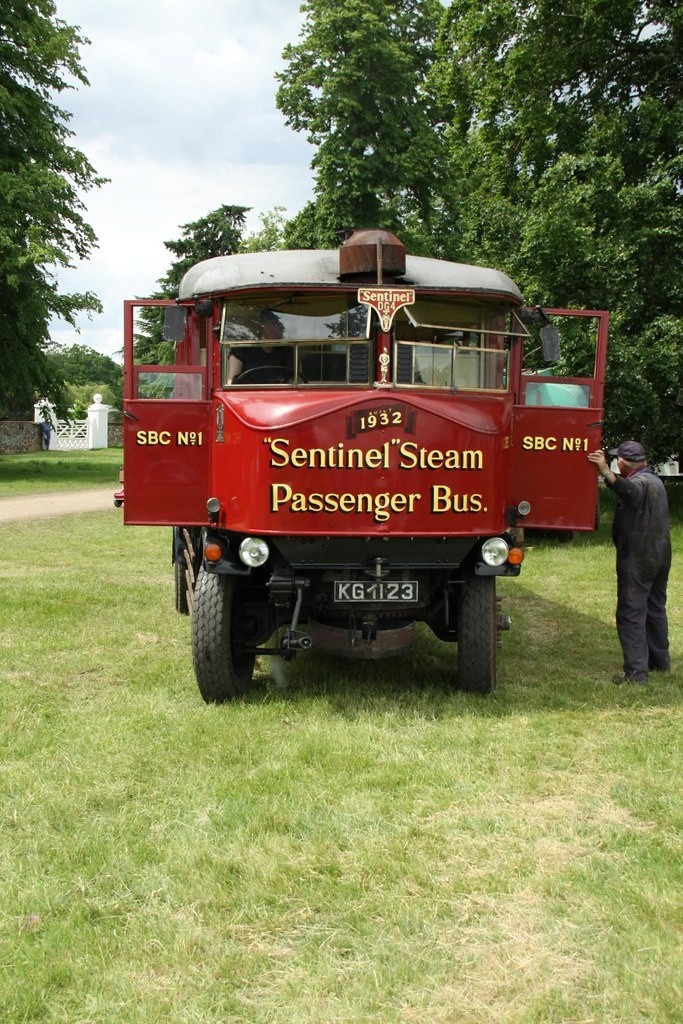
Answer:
[{"left": 123, "top": 229, "right": 609, "bottom": 703}]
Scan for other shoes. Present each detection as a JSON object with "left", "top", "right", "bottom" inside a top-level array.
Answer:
[{"left": 612, "top": 672, "right": 648, "bottom": 686}]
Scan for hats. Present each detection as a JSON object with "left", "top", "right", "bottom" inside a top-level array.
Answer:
[{"left": 608, "top": 441, "right": 646, "bottom": 462}]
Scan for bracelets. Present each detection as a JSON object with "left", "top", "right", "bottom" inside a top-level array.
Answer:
[{"left": 602, "top": 468, "right": 612, "bottom": 478}]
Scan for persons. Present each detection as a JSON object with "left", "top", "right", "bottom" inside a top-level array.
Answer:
[
  {"left": 226, "top": 305, "right": 305, "bottom": 385},
  {"left": 586, "top": 440, "right": 672, "bottom": 685},
  {"left": 29, "top": 416, "right": 54, "bottom": 451}
]
